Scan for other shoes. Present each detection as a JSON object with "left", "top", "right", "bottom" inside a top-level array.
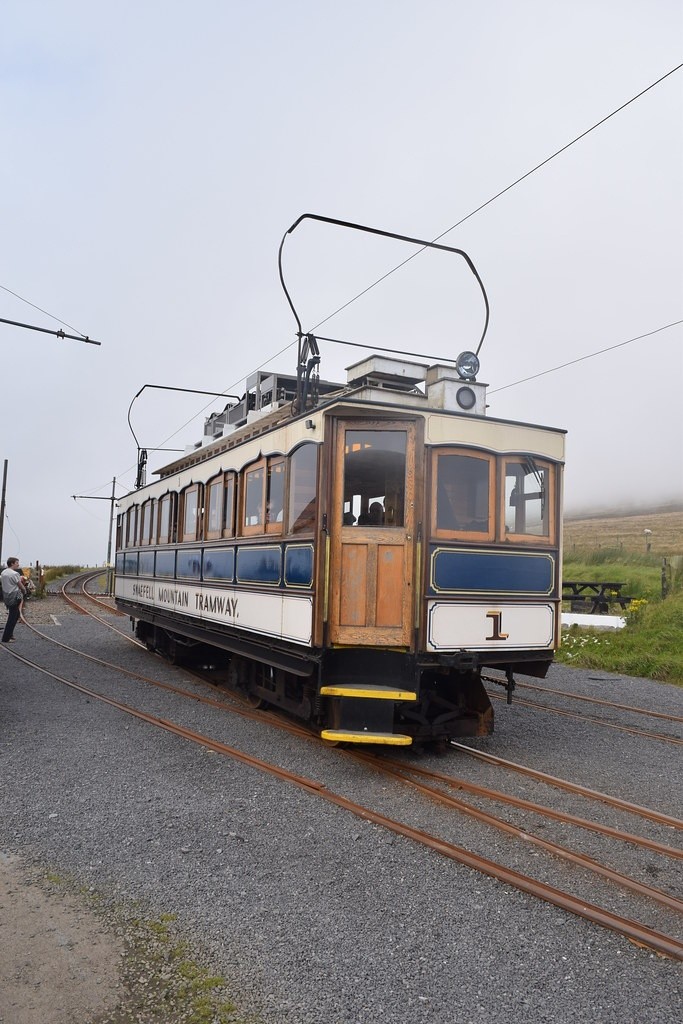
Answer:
[{"left": 7, "top": 635, "right": 16, "bottom": 643}]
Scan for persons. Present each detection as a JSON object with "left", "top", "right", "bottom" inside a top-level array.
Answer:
[
  {"left": 1, "top": 557, "right": 27, "bottom": 643},
  {"left": 170, "top": 501, "right": 385, "bottom": 544}
]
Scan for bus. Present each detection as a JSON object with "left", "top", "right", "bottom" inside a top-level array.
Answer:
[{"left": 110, "top": 352, "right": 567, "bottom": 757}]
